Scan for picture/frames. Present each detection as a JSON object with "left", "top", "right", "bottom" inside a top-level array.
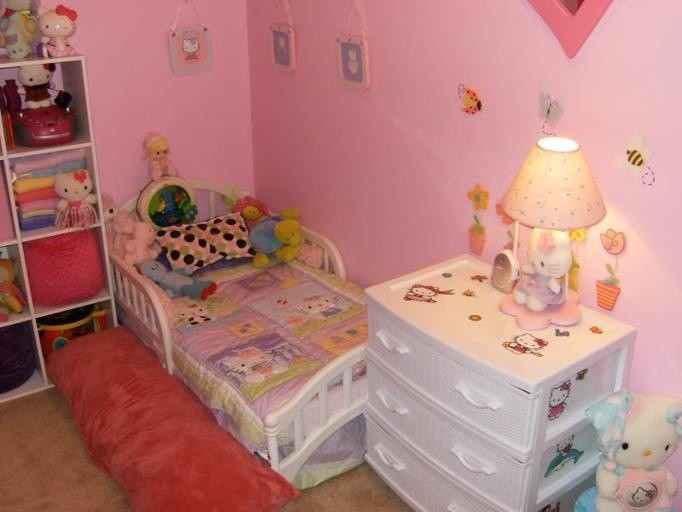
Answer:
[
  {"left": 527, "top": 0, "right": 612, "bottom": 59},
  {"left": 267, "top": 23, "right": 296, "bottom": 72},
  {"left": 338, "top": 35, "right": 370, "bottom": 90},
  {"left": 170, "top": 25, "right": 212, "bottom": 74}
]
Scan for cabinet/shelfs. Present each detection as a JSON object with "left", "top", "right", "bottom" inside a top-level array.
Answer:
[
  {"left": 364, "top": 254, "right": 637, "bottom": 512},
  {"left": 0, "top": 53, "right": 118, "bottom": 404}
]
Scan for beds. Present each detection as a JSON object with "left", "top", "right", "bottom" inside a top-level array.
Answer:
[{"left": 104, "top": 178, "right": 368, "bottom": 484}]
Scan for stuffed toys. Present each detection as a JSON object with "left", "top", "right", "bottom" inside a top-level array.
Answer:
[
  {"left": 110, "top": 209, "right": 162, "bottom": 267},
  {"left": 53, "top": 167, "right": 99, "bottom": 230},
  {"left": 230, "top": 194, "right": 268, "bottom": 234},
  {"left": 585, "top": 386, "right": 682, "bottom": 512},
  {"left": 143, "top": 132, "right": 179, "bottom": 184},
  {"left": 0, "top": 0, "right": 39, "bottom": 60},
  {"left": 249, "top": 207, "right": 303, "bottom": 270},
  {"left": 140, "top": 257, "right": 216, "bottom": 300},
  {"left": 0, "top": 257, "right": 27, "bottom": 315},
  {"left": 513, "top": 224, "right": 574, "bottom": 313},
  {"left": 15, "top": 64, "right": 57, "bottom": 110}
]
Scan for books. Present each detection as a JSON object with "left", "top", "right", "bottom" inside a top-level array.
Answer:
[
  {"left": 4, "top": 111, "right": 13, "bottom": 150},
  {"left": 1, "top": 113, "right": 9, "bottom": 151},
  {"left": 7, "top": 111, "right": 16, "bottom": 150}
]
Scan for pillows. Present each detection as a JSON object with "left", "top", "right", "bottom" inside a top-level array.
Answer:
[
  {"left": 46, "top": 324, "right": 301, "bottom": 512},
  {"left": 155, "top": 210, "right": 255, "bottom": 276}
]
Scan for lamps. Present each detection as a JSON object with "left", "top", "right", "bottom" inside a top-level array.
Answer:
[{"left": 499, "top": 136, "right": 607, "bottom": 332}]
